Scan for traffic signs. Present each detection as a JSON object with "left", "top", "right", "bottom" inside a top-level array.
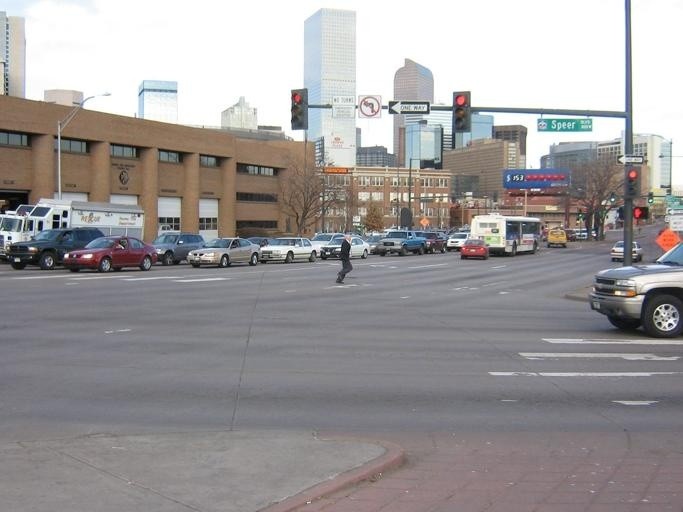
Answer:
[
  {"left": 667, "top": 209, "right": 683, "bottom": 216},
  {"left": 389, "top": 99, "right": 431, "bottom": 116},
  {"left": 331, "top": 94, "right": 355, "bottom": 119},
  {"left": 537, "top": 118, "right": 594, "bottom": 134},
  {"left": 616, "top": 155, "right": 645, "bottom": 167}
]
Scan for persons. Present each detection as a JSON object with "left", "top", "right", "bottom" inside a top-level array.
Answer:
[
  {"left": 116, "top": 241, "right": 124, "bottom": 249},
  {"left": 334, "top": 234, "right": 352, "bottom": 285},
  {"left": 231, "top": 240, "right": 239, "bottom": 248}
]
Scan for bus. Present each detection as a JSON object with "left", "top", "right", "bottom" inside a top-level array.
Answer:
[{"left": 466, "top": 213, "right": 545, "bottom": 257}]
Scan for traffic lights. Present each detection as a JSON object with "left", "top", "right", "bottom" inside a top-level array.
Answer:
[
  {"left": 647, "top": 191, "right": 655, "bottom": 207},
  {"left": 633, "top": 205, "right": 652, "bottom": 221},
  {"left": 452, "top": 90, "right": 475, "bottom": 134},
  {"left": 290, "top": 87, "right": 310, "bottom": 132},
  {"left": 610, "top": 192, "right": 617, "bottom": 204},
  {"left": 624, "top": 166, "right": 642, "bottom": 201},
  {"left": 618, "top": 206, "right": 625, "bottom": 221}
]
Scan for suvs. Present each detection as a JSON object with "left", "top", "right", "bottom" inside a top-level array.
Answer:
[
  {"left": 6, "top": 228, "right": 106, "bottom": 271},
  {"left": 150, "top": 233, "right": 206, "bottom": 266}
]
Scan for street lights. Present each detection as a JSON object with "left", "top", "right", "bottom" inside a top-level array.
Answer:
[
  {"left": 54, "top": 90, "right": 116, "bottom": 203},
  {"left": 408, "top": 157, "right": 434, "bottom": 222},
  {"left": 657, "top": 141, "right": 674, "bottom": 198}
]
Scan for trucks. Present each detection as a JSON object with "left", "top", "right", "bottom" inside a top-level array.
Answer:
[{"left": 0, "top": 204, "right": 145, "bottom": 262}]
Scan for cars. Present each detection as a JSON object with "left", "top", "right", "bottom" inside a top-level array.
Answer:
[
  {"left": 460, "top": 239, "right": 491, "bottom": 261},
  {"left": 311, "top": 227, "right": 470, "bottom": 260},
  {"left": 187, "top": 237, "right": 262, "bottom": 269},
  {"left": 542, "top": 223, "right": 606, "bottom": 249},
  {"left": 260, "top": 237, "right": 322, "bottom": 264},
  {"left": 588, "top": 239, "right": 683, "bottom": 344},
  {"left": 609, "top": 239, "right": 645, "bottom": 265},
  {"left": 247, "top": 236, "right": 274, "bottom": 249},
  {"left": 63, "top": 235, "right": 158, "bottom": 274}
]
